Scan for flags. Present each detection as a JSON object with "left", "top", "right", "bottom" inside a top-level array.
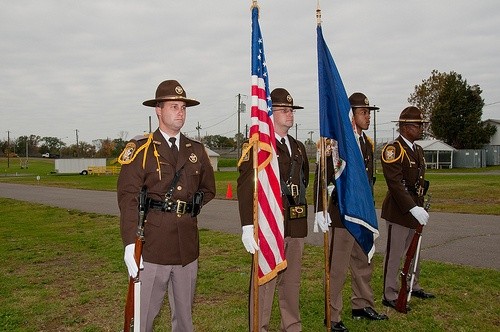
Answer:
[
  {"left": 316, "top": 22, "right": 380, "bottom": 266},
  {"left": 249, "top": 3, "right": 288, "bottom": 287}
]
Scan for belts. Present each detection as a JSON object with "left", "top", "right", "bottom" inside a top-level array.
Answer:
[
  {"left": 145, "top": 198, "right": 193, "bottom": 214},
  {"left": 282, "top": 184, "right": 300, "bottom": 196},
  {"left": 404, "top": 186, "right": 423, "bottom": 197}
]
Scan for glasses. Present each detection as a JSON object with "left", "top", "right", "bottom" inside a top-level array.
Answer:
[
  {"left": 273, "top": 108, "right": 296, "bottom": 114},
  {"left": 405, "top": 123, "right": 423, "bottom": 128}
]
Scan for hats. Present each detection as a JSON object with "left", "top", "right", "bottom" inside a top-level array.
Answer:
[
  {"left": 143, "top": 80, "right": 200, "bottom": 107},
  {"left": 391, "top": 107, "right": 431, "bottom": 123},
  {"left": 271, "top": 88, "right": 305, "bottom": 109},
  {"left": 349, "top": 91, "right": 380, "bottom": 110}
]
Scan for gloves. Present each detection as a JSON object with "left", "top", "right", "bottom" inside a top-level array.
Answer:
[
  {"left": 409, "top": 206, "right": 429, "bottom": 226},
  {"left": 241, "top": 225, "right": 259, "bottom": 254},
  {"left": 314, "top": 211, "right": 332, "bottom": 233},
  {"left": 124, "top": 243, "right": 144, "bottom": 278}
]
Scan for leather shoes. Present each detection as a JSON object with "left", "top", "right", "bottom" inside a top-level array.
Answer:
[
  {"left": 407, "top": 289, "right": 436, "bottom": 298},
  {"left": 352, "top": 307, "right": 389, "bottom": 321},
  {"left": 382, "top": 299, "right": 411, "bottom": 311},
  {"left": 324, "top": 319, "right": 349, "bottom": 332}
]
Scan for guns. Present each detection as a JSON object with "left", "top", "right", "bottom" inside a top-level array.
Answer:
[
  {"left": 394, "top": 192, "right": 433, "bottom": 314},
  {"left": 123, "top": 211, "right": 146, "bottom": 332}
]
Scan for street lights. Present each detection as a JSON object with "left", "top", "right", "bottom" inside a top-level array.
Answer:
[{"left": 374, "top": 105, "right": 380, "bottom": 175}]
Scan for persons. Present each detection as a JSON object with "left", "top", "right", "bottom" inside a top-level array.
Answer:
[
  {"left": 380, "top": 106, "right": 437, "bottom": 313},
  {"left": 313, "top": 93, "right": 390, "bottom": 332},
  {"left": 116, "top": 79, "right": 217, "bottom": 332},
  {"left": 236, "top": 87, "right": 310, "bottom": 332}
]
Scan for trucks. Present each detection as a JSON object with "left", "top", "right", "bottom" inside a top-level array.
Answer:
[{"left": 42, "top": 153, "right": 56, "bottom": 158}]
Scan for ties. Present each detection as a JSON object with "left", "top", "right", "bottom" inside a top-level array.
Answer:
[
  {"left": 413, "top": 144, "right": 419, "bottom": 160},
  {"left": 169, "top": 137, "right": 178, "bottom": 158},
  {"left": 359, "top": 137, "right": 366, "bottom": 159},
  {"left": 281, "top": 138, "right": 290, "bottom": 158}
]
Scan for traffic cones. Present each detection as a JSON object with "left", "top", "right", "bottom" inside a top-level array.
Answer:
[{"left": 226, "top": 183, "right": 233, "bottom": 199}]
